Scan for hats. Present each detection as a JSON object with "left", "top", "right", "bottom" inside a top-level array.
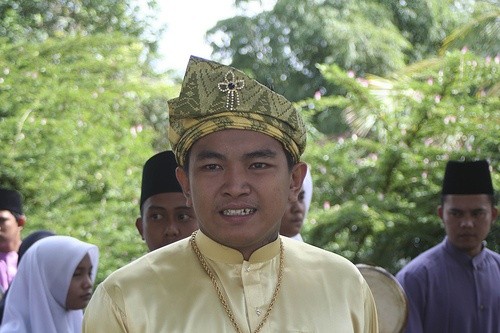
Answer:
[
  {"left": 140, "top": 150, "right": 182, "bottom": 210},
  {"left": 167, "top": 55, "right": 307, "bottom": 166},
  {"left": 442, "top": 160, "right": 494, "bottom": 195},
  {"left": 0, "top": 189, "right": 22, "bottom": 215}
]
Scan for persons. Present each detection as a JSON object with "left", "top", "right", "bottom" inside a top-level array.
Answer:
[
  {"left": 136, "top": 150, "right": 199, "bottom": 251},
  {"left": 279, "top": 165, "right": 313, "bottom": 242},
  {"left": 395, "top": 159, "right": 500, "bottom": 333},
  {"left": 82, "top": 55, "right": 379, "bottom": 333},
  {"left": 0, "top": 189, "right": 99, "bottom": 333}
]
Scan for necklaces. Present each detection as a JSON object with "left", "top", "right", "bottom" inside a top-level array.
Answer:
[{"left": 190, "top": 229, "right": 284, "bottom": 333}]
